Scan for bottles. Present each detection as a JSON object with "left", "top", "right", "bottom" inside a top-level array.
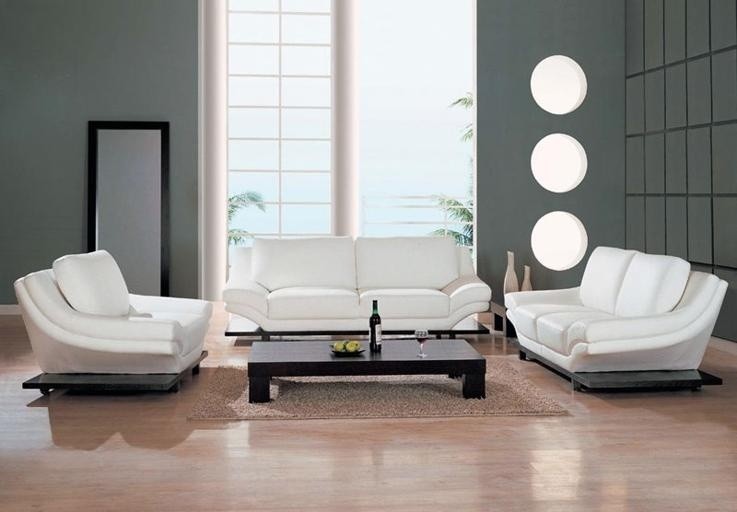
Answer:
[{"left": 369, "top": 300, "right": 382, "bottom": 353}]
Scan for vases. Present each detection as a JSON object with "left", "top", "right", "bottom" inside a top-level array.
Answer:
[
  {"left": 503, "top": 250, "right": 519, "bottom": 295},
  {"left": 521, "top": 265, "right": 533, "bottom": 291}
]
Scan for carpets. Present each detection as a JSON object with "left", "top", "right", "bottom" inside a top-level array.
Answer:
[{"left": 186, "top": 353, "right": 573, "bottom": 421}]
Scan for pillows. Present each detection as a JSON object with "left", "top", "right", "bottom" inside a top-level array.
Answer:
[
  {"left": 353, "top": 236, "right": 459, "bottom": 290},
  {"left": 579, "top": 245, "right": 639, "bottom": 314},
  {"left": 251, "top": 236, "right": 357, "bottom": 290},
  {"left": 613, "top": 252, "right": 691, "bottom": 318},
  {"left": 53, "top": 249, "right": 130, "bottom": 316}
]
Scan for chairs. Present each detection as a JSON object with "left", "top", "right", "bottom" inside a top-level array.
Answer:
[{"left": 13, "top": 268, "right": 214, "bottom": 397}]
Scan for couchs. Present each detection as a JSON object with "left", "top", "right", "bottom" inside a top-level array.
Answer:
[
  {"left": 220, "top": 245, "right": 493, "bottom": 341},
  {"left": 503, "top": 270, "right": 729, "bottom": 392}
]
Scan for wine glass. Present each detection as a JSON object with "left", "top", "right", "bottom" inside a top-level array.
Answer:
[{"left": 415, "top": 329, "right": 428, "bottom": 358}]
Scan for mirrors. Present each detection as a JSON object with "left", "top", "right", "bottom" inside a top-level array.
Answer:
[{"left": 86, "top": 120, "right": 171, "bottom": 296}]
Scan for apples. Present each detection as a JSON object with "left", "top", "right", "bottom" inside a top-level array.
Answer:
[{"left": 334, "top": 340, "right": 360, "bottom": 352}]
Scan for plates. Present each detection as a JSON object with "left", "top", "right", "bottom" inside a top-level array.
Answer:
[{"left": 330, "top": 350, "right": 367, "bottom": 356}]
